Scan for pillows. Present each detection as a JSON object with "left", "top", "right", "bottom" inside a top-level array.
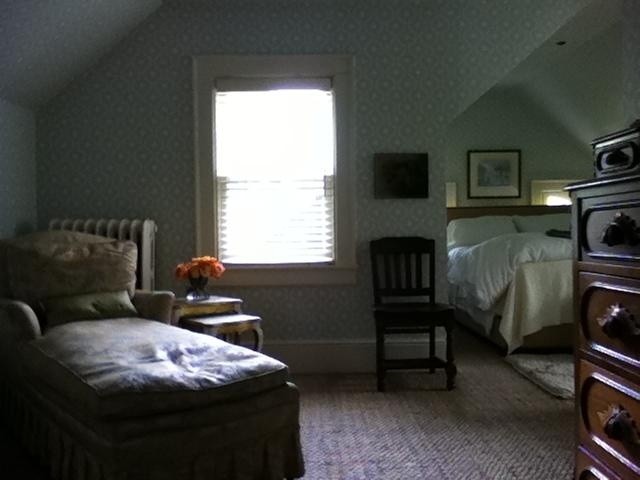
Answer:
[
  {"left": 514, "top": 213, "right": 570, "bottom": 232},
  {"left": 40, "top": 291, "right": 139, "bottom": 326},
  {"left": 7, "top": 239, "right": 138, "bottom": 299},
  {"left": 448, "top": 216, "right": 517, "bottom": 248}
]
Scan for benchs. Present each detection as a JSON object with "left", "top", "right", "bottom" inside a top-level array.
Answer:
[{"left": 0, "top": 230, "right": 300, "bottom": 480}]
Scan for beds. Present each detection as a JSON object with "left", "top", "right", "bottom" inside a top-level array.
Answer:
[{"left": 448, "top": 208, "right": 574, "bottom": 357}]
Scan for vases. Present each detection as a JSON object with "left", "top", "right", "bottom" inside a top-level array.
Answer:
[{"left": 184, "top": 277, "right": 210, "bottom": 302}]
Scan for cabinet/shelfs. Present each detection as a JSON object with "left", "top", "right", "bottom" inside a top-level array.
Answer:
[{"left": 563, "top": 124, "right": 640, "bottom": 480}]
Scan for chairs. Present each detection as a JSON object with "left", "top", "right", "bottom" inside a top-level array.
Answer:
[{"left": 369, "top": 236, "right": 457, "bottom": 393}]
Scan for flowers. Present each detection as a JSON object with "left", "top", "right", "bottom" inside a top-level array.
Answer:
[{"left": 175, "top": 255, "right": 226, "bottom": 280}]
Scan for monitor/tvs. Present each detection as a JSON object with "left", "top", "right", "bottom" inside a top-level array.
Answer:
[{"left": 373, "top": 152, "right": 429, "bottom": 199}]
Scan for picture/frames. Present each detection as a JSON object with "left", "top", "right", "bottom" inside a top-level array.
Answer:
[{"left": 467, "top": 149, "right": 521, "bottom": 199}]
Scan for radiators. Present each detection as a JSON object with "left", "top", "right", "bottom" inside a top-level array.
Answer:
[{"left": 48, "top": 218, "right": 158, "bottom": 290}]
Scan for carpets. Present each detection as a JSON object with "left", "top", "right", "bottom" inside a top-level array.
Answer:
[{"left": 504, "top": 353, "right": 576, "bottom": 400}]
